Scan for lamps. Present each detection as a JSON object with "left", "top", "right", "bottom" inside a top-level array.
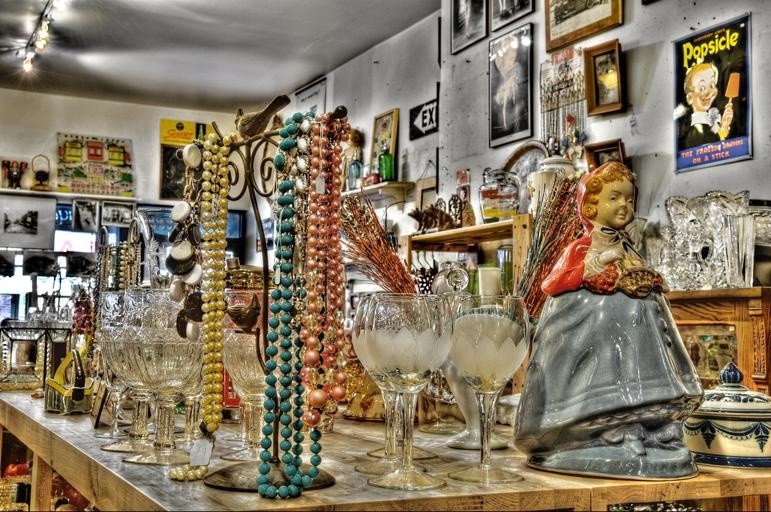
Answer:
[{"left": 17, "top": 0, "right": 68, "bottom": 74}]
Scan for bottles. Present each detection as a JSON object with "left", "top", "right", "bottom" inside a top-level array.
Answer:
[
  {"left": 378, "top": 141, "right": 394, "bottom": 181},
  {"left": 346, "top": 148, "right": 363, "bottom": 189},
  {"left": 198, "top": 125, "right": 204, "bottom": 141}
]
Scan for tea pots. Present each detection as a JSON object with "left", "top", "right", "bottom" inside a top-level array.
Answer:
[{"left": 477, "top": 166, "right": 522, "bottom": 223}]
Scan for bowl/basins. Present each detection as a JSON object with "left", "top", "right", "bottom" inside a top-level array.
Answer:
[{"left": 8, "top": 306, "right": 73, "bottom": 342}]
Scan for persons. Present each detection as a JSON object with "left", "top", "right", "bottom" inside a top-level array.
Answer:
[
  {"left": 683, "top": 59, "right": 733, "bottom": 144},
  {"left": 518, "top": 159, "right": 698, "bottom": 483}
]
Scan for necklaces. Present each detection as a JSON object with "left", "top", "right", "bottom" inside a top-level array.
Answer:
[
  {"left": 255, "top": 106, "right": 354, "bottom": 498},
  {"left": 87, "top": 240, "right": 138, "bottom": 357},
  {"left": 168, "top": 130, "right": 233, "bottom": 483}
]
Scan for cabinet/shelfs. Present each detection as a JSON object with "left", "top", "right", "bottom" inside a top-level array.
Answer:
[
  {"left": 405, "top": 213, "right": 534, "bottom": 309},
  {"left": 652, "top": 284, "right": 771, "bottom": 409}
]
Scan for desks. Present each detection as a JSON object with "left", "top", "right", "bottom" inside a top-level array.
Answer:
[{"left": 1, "top": 392, "right": 771, "bottom": 512}]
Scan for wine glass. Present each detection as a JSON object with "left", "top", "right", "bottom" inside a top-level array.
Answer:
[
  {"left": 347, "top": 293, "right": 531, "bottom": 495},
  {"left": 90, "top": 285, "right": 279, "bottom": 467}
]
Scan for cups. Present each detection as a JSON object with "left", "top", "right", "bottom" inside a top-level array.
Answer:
[
  {"left": 640, "top": 189, "right": 771, "bottom": 303},
  {"left": 479, "top": 268, "right": 501, "bottom": 295}
]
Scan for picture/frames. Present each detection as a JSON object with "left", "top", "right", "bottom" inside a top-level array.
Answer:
[
  {"left": 584, "top": 138, "right": 624, "bottom": 182},
  {"left": 672, "top": 12, "right": 755, "bottom": 174},
  {"left": 415, "top": 176, "right": 436, "bottom": 212},
  {"left": 366, "top": 108, "right": 400, "bottom": 182},
  {"left": 55, "top": 201, "right": 244, "bottom": 255},
  {"left": 456, "top": 183, "right": 471, "bottom": 205},
  {"left": 487, "top": 0, "right": 536, "bottom": 32},
  {"left": 449, "top": 0, "right": 487, "bottom": 56},
  {"left": 544, "top": 0, "right": 625, "bottom": 54},
  {"left": 488, "top": 22, "right": 536, "bottom": 149},
  {"left": 584, "top": 39, "right": 628, "bottom": 118}
]
